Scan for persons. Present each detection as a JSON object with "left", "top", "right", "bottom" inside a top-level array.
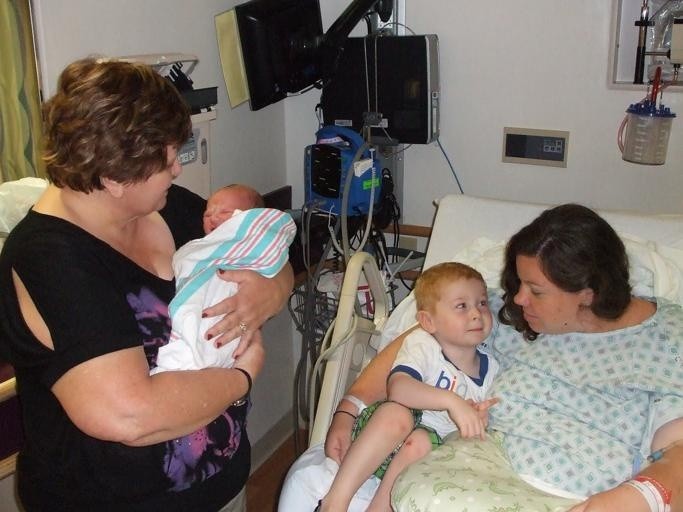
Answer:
[
  {"left": 313, "top": 260, "right": 499, "bottom": 510},
  {"left": 1, "top": 55, "right": 298, "bottom": 511},
  {"left": 153, "top": 182, "right": 296, "bottom": 374}
]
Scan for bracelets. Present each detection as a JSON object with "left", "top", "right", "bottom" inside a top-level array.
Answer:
[
  {"left": 637, "top": 472, "right": 674, "bottom": 511},
  {"left": 332, "top": 408, "right": 357, "bottom": 420},
  {"left": 231, "top": 364, "right": 252, "bottom": 412}
]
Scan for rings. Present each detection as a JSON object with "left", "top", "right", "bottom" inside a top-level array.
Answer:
[{"left": 239, "top": 319, "right": 248, "bottom": 331}]
